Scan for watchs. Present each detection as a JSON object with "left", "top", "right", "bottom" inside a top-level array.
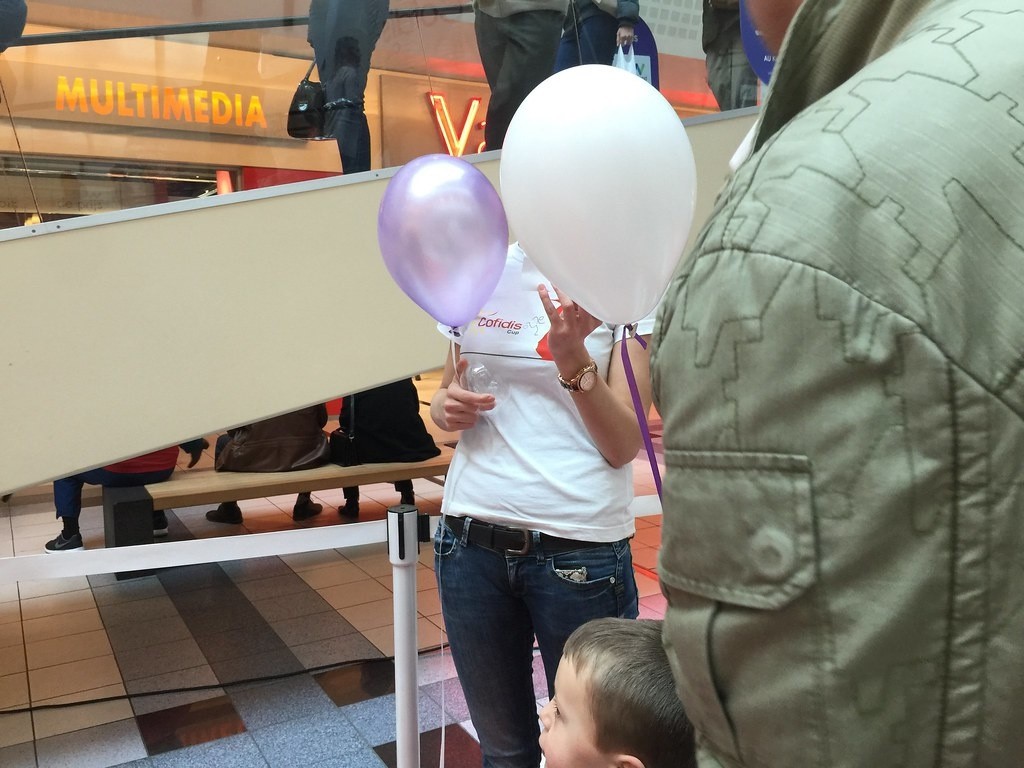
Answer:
[{"left": 557, "top": 355, "right": 599, "bottom": 394}]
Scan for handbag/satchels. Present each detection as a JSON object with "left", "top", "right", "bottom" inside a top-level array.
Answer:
[
  {"left": 614, "top": 41, "right": 635, "bottom": 74},
  {"left": 287, "top": 58, "right": 325, "bottom": 140},
  {"left": 327, "top": 427, "right": 362, "bottom": 467},
  {"left": 592, "top": 0, "right": 618, "bottom": 18}
]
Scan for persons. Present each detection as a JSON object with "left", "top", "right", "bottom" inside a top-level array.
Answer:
[
  {"left": 308, "top": 0, "right": 390, "bottom": 176},
  {"left": 44, "top": 438, "right": 210, "bottom": 554},
  {"left": 430, "top": 239, "right": 674, "bottom": 768},
  {"left": 538, "top": 617, "right": 697, "bottom": 768},
  {"left": 328, "top": 377, "right": 441, "bottom": 521},
  {"left": 554, "top": 0, "right": 640, "bottom": 73},
  {"left": 206, "top": 402, "right": 330, "bottom": 525},
  {"left": 702, "top": 0, "right": 758, "bottom": 112},
  {"left": 644, "top": 0, "right": 1024, "bottom": 768},
  {"left": 472, "top": 0, "right": 572, "bottom": 152}
]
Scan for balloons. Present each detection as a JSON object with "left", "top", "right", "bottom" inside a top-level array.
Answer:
[
  {"left": 498, "top": 63, "right": 698, "bottom": 338},
  {"left": 378, "top": 153, "right": 510, "bottom": 337}
]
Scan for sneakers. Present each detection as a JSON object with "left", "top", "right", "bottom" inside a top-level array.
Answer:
[
  {"left": 206, "top": 504, "right": 244, "bottom": 524},
  {"left": 293, "top": 500, "right": 324, "bottom": 520},
  {"left": 153, "top": 516, "right": 170, "bottom": 536},
  {"left": 44, "top": 529, "right": 84, "bottom": 553}
]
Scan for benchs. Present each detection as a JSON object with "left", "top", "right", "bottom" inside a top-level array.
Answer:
[{"left": 102, "top": 440, "right": 456, "bottom": 579}]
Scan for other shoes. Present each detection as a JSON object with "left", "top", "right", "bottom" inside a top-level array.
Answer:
[
  {"left": 400, "top": 491, "right": 415, "bottom": 505},
  {"left": 188, "top": 438, "right": 210, "bottom": 469},
  {"left": 337, "top": 499, "right": 359, "bottom": 517}
]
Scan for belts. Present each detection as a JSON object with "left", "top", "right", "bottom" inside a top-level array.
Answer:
[{"left": 441, "top": 514, "right": 611, "bottom": 554}]
